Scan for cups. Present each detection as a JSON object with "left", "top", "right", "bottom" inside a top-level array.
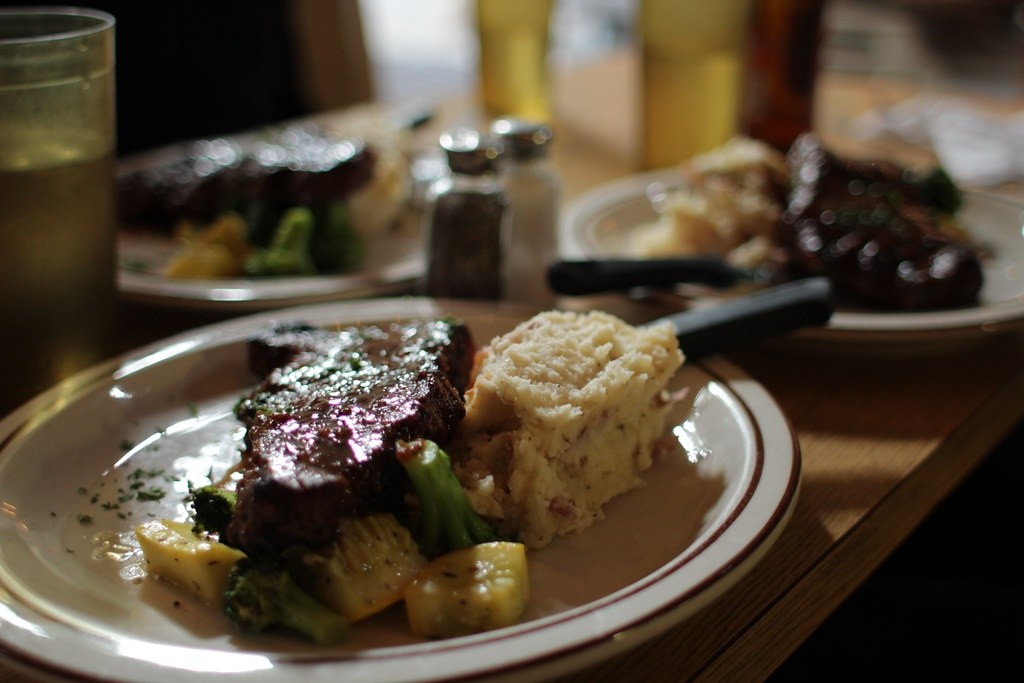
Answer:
[
  {"left": 743, "top": 0, "right": 825, "bottom": 161},
  {"left": 0, "top": 6, "right": 124, "bottom": 294},
  {"left": 477, "top": 0, "right": 552, "bottom": 126},
  {"left": 633, "top": 2, "right": 743, "bottom": 168}
]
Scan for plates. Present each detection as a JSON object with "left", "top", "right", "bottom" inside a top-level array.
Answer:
[
  {"left": 552, "top": 163, "right": 1024, "bottom": 360},
  {"left": 0, "top": 297, "right": 802, "bottom": 683},
  {"left": 113, "top": 130, "right": 422, "bottom": 311}
]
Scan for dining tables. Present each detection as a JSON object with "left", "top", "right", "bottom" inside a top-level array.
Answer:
[{"left": 0, "top": 51, "right": 1024, "bottom": 683}]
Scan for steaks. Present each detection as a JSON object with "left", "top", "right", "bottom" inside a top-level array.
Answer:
[
  {"left": 230, "top": 317, "right": 477, "bottom": 539},
  {"left": 114, "top": 123, "right": 376, "bottom": 229},
  {"left": 778, "top": 135, "right": 984, "bottom": 311}
]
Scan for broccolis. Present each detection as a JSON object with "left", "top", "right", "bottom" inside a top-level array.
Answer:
[
  {"left": 191, "top": 438, "right": 522, "bottom": 648},
  {"left": 216, "top": 198, "right": 366, "bottom": 280}
]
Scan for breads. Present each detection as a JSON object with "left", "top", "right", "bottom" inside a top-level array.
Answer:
[
  {"left": 457, "top": 311, "right": 689, "bottom": 549},
  {"left": 626, "top": 139, "right": 792, "bottom": 280}
]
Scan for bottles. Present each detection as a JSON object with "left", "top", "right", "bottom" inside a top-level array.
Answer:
[
  {"left": 484, "top": 121, "right": 563, "bottom": 300},
  {"left": 422, "top": 132, "right": 505, "bottom": 304}
]
